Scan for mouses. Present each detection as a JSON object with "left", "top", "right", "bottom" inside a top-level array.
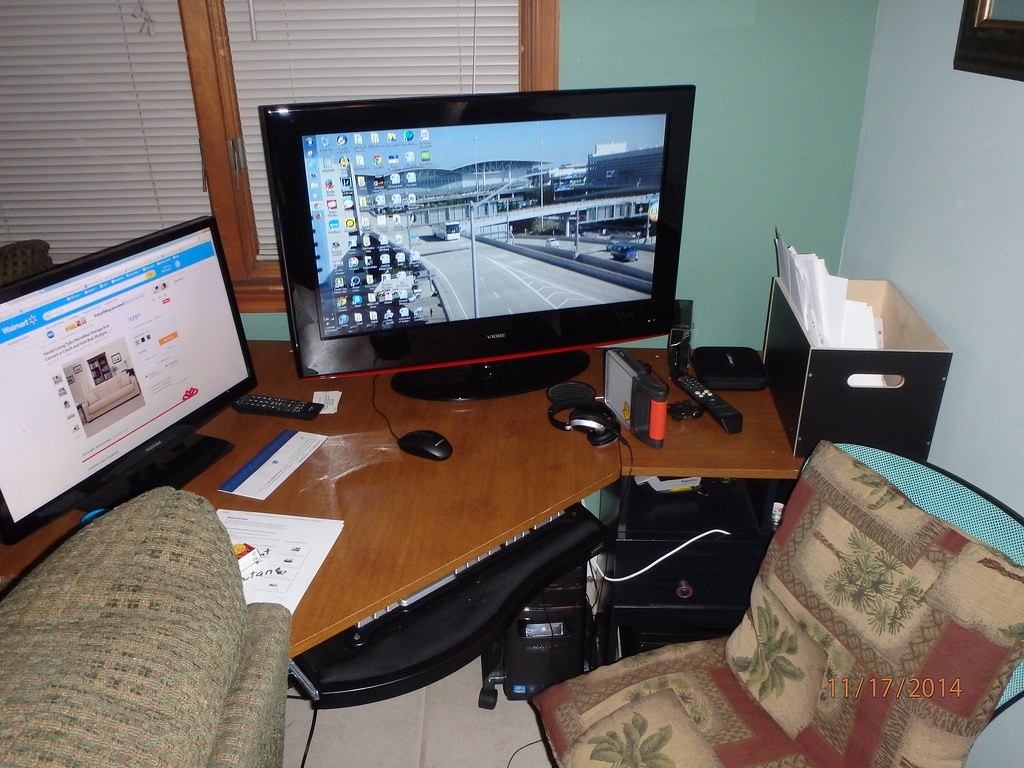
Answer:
[{"left": 397, "top": 430, "right": 453, "bottom": 461}]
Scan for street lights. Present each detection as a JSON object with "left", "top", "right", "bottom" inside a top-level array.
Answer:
[{"left": 469, "top": 167, "right": 559, "bottom": 318}]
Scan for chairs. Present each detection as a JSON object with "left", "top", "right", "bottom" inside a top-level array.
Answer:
[{"left": 532, "top": 440, "right": 1024, "bottom": 768}]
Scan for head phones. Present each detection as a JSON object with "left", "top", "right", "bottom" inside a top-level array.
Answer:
[{"left": 548, "top": 399, "right": 622, "bottom": 448}]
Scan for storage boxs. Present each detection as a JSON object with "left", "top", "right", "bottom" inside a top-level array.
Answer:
[{"left": 763, "top": 277, "right": 953, "bottom": 466}]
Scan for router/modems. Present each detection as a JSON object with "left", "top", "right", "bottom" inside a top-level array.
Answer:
[{"left": 692, "top": 346, "right": 768, "bottom": 392}]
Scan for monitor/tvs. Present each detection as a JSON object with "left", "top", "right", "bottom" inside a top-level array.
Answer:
[
  {"left": 257, "top": 84, "right": 696, "bottom": 400},
  {"left": 0, "top": 215, "right": 258, "bottom": 547}
]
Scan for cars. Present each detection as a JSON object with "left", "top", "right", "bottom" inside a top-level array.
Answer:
[
  {"left": 545, "top": 237, "right": 560, "bottom": 248},
  {"left": 606, "top": 240, "right": 621, "bottom": 251}
]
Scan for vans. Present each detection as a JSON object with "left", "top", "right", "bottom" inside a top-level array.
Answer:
[{"left": 610, "top": 241, "right": 640, "bottom": 261}]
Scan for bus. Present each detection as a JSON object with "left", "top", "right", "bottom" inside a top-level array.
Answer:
[{"left": 431, "top": 220, "right": 462, "bottom": 240}]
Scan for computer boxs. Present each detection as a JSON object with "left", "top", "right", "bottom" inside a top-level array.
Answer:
[{"left": 503, "top": 562, "right": 587, "bottom": 700}]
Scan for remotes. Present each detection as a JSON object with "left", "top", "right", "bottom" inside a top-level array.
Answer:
[
  {"left": 232, "top": 394, "right": 325, "bottom": 421},
  {"left": 675, "top": 373, "right": 744, "bottom": 434}
]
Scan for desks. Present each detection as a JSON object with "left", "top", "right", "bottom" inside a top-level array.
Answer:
[{"left": 0, "top": 340, "right": 806, "bottom": 666}]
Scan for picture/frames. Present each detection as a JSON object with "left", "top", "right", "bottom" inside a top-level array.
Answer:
[{"left": 953, "top": 0, "right": 1024, "bottom": 82}]
